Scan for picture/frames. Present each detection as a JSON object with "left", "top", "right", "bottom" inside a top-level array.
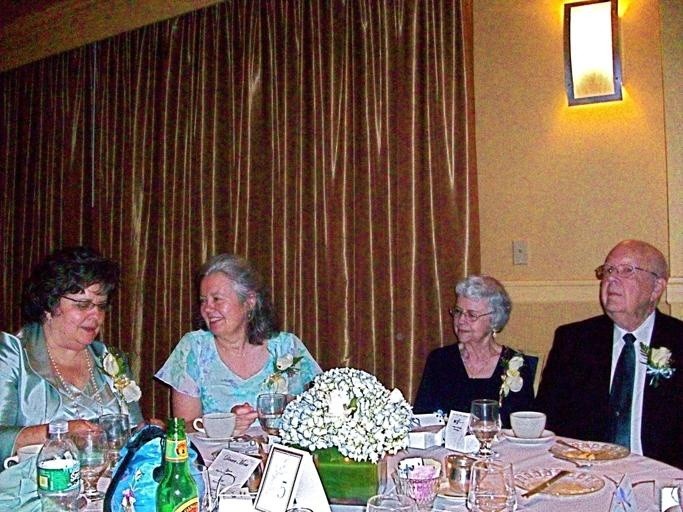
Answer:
[{"left": 563, "top": 0, "right": 623, "bottom": 106}]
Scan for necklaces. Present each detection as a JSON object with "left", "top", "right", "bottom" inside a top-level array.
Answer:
[
  {"left": 465, "top": 361, "right": 488, "bottom": 379},
  {"left": 47, "top": 347, "right": 103, "bottom": 421}
]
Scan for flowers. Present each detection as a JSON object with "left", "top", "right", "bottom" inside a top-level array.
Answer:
[
  {"left": 275, "top": 349, "right": 304, "bottom": 374},
  {"left": 104, "top": 346, "right": 142, "bottom": 404},
  {"left": 498, "top": 355, "right": 524, "bottom": 405},
  {"left": 639, "top": 341, "right": 676, "bottom": 385},
  {"left": 278, "top": 368, "right": 414, "bottom": 463}
]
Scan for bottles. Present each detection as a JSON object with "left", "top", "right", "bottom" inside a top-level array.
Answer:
[
  {"left": 35, "top": 417, "right": 80, "bottom": 512},
  {"left": 154, "top": 416, "right": 201, "bottom": 512}
]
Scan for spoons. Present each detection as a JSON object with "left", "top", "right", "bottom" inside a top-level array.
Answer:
[{"left": 553, "top": 455, "right": 593, "bottom": 469}]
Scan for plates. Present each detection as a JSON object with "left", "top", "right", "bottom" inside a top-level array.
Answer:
[
  {"left": 435, "top": 481, "right": 467, "bottom": 502},
  {"left": 501, "top": 428, "right": 556, "bottom": 446},
  {"left": 193, "top": 432, "right": 235, "bottom": 441},
  {"left": 547, "top": 439, "right": 631, "bottom": 464},
  {"left": 512, "top": 468, "right": 606, "bottom": 496}
]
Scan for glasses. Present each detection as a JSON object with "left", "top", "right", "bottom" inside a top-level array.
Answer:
[
  {"left": 60, "top": 296, "right": 110, "bottom": 312},
  {"left": 449, "top": 306, "right": 495, "bottom": 323},
  {"left": 595, "top": 264, "right": 658, "bottom": 280}
]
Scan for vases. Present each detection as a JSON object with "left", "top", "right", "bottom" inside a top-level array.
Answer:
[{"left": 315, "top": 447, "right": 379, "bottom": 505}]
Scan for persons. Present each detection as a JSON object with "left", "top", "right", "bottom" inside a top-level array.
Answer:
[
  {"left": 535, "top": 239, "right": 683, "bottom": 472},
  {"left": 154, "top": 254, "right": 324, "bottom": 433},
  {"left": 0, "top": 246, "right": 167, "bottom": 473},
  {"left": 412, "top": 274, "right": 534, "bottom": 429}
]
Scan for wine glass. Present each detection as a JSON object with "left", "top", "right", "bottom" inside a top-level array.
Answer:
[
  {"left": 469, "top": 398, "right": 503, "bottom": 462},
  {"left": 71, "top": 412, "right": 131, "bottom": 505}
]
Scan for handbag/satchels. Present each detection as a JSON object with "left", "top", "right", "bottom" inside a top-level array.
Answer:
[{"left": 102, "top": 425, "right": 205, "bottom": 510}]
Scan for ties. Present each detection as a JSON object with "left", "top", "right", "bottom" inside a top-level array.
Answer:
[{"left": 609, "top": 333, "right": 636, "bottom": 452}]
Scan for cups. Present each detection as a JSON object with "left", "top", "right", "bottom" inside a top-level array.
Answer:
[
  {"left": 445, "top": 453, "right": 480, "bottom": 493},
  {"left": 364, "top": 492, "right": 419, "bottom": 512},
  {"left": 390, "top": 470, "right": 444, "bottom": 512},
  {"left": 256, "top": 392, "right": 286, "bottom": 435},
  {"left": 193, "top": 411, "right": 237, "bottom": 438},
  {"left": 509, "top": 410, "right": 547, "bottom": 438},
  {"left": 3, "top": 444, "right": 44, "bottom": 470},
  {"left": 465, "top": 458, "right": 518, "bottom": 512}
]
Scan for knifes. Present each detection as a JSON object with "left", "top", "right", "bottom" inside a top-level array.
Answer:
[{"left": 519, "top": 470, "right": 570, "bottom": 498}]
